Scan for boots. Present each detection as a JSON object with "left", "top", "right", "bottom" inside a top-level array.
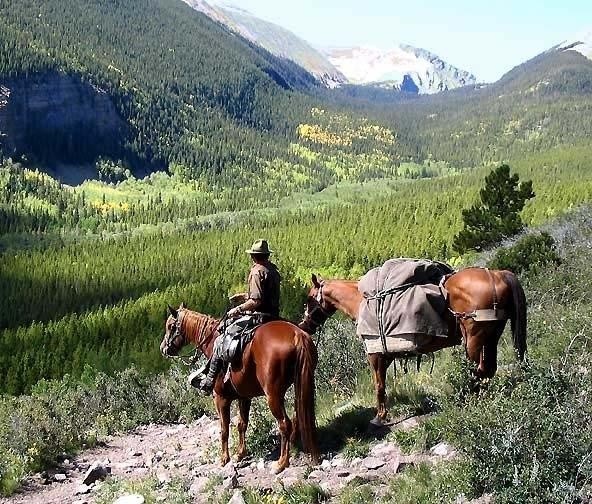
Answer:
[{"left": 192, "top": 356, "right": 222, "bottom": 395}]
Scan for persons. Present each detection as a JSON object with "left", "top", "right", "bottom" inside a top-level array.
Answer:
[{"left": 193, "top": 239, "right": 283, "bottom": 396}]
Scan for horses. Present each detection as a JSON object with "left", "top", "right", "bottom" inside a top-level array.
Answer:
[
  {"left": 159, "top": 300, "right": 318, "bottom": 473},
  {"left": 297, "top": 266, "right": 527, "bottom": 421}
]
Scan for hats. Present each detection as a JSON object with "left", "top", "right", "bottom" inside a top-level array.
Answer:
[{"left": 245, "top": 239, "right": 275, "bottom": 254}]
